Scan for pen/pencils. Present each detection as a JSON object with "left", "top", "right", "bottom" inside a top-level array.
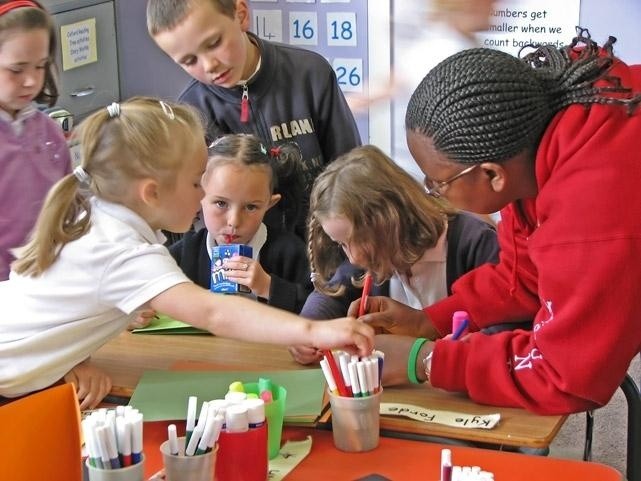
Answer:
[
  {"left": 453, "top": 320, "right": 468, "bottom": 340},
  {"left": 154, "top": 315, "right": 160, "bottom": 320},
  {"left": 358, "top": 272, "right": 372, "bottom": 318},
  {"left": 441, "top": 448, "right": 495, "bottom": 481},
  {"left": 319, "top": 347, "right": 384, "bottom": 452},
  {"left": 167, "top": 376, "right": 274, "bottom": 481},
  {"left": 81, "top": 405, "right": 144, "bottom": 481}
]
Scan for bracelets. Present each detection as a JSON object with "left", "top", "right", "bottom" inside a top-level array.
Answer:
[{"left": 406, "top": 335, "right": 428, "bottom": 387}]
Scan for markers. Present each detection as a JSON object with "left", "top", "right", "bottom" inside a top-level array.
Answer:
[{"left": 453, "top": 311, "right": 469, "bottom": 341}]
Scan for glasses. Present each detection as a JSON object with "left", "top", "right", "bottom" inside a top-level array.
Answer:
[{"left": 422, "top": 164, "right": 478, "bottom": 199}]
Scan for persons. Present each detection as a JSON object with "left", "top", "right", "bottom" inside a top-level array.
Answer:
[
  {"left": 351, "top": 21, "right": 641, "bottom": 415},
  {"left": 125, "top": 132, "right": 320, "bottom": 331},
  {"left": 0, "top": 0, "right": 82, "bottom": 280},
  {"left": 404, "top": 0, "right": 495, "bottom": 111},
  {"left": 144, "top": 0, "right": 360, "bottom": 239},
  {"left": 0, "top": 95, "right": 374, "bottom": 410},
  {"left": 288, "top": 144, "right": 532, "bottom": 367}
]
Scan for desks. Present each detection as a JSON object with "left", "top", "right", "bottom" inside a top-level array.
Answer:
[
  {"left": 62, "top": 330, "right": 595, "bottom": 463},
  {"left": 80, "top": 398, "right": 622, "bottom": 481}
]
